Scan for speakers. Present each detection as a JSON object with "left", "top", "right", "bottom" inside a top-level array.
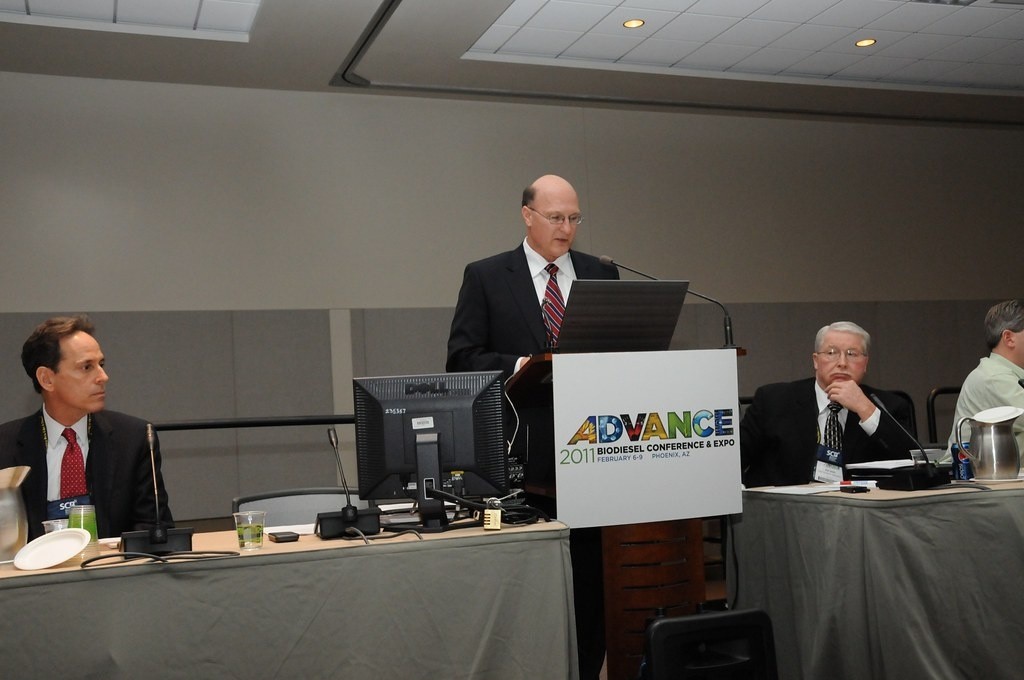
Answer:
[{"left": 644, "top": 601, "right": 778, "bottom": 680}]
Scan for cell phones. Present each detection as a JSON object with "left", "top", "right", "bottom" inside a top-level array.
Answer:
[
  {"left": 1018, "top": 378, "right": 1024, "bottom": 389},
  {"left": 269, "top": 532, "right": 300, "bottom": 542},
  {"left": 840, "top": 485, "right": 868, "bottom": 494}
]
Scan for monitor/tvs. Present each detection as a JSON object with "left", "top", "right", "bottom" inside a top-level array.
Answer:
[{"left": 353, "top": 370, "right": 510, "bottom": 533}]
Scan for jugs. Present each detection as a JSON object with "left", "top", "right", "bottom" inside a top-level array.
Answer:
[
  {"left": 955, "top": 406, "right": 1023, "bottom": 480},
  {"left": 0, "top": 466, "right": 32, "bottom": 564}
]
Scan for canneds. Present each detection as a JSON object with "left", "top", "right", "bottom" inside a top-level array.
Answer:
[{"left": 951, "top": 441, "right": 974, "bottom": 480}]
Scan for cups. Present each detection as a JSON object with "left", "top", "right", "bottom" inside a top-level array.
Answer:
[
  {"left": 233, "top": 511, "right": 267, "bottom": 550},
  {"left": 41, "top": 518, "right": 66, "bottom": 534},
  {"left": 910, "top": 449, "right": 940, "bottom": 461},
  {"left": 67, "top": 504, "right": 100, "bottom": 559}
]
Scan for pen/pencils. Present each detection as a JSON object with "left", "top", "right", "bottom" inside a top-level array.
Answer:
[{"left": 833, "top": 481, "right": 877, "bottom": 485}]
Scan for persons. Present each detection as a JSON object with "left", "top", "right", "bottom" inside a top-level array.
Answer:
[
  {"left": 740, "top": 321, "right": 904, "bottom": 488},
  {"left": 0, "top": 317, "right": 174, "bottom": 544},
  {"left": 445, "top": 175, "right": 618, "bottom": 680},
  {"left": 939, "top": 301, "right": 1024, "bottom": 461}
]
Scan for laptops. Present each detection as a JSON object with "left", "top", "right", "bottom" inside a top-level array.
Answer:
[{"left": 552, "top": 281, "right": 690, "bottom": 354}]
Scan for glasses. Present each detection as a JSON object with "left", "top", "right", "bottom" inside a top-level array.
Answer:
[
  {"left": 527, "top": 207, "right": 585, "bottom": 225},
  {"left": 816, "top": 348, "right": 867, "bottom": 360}
]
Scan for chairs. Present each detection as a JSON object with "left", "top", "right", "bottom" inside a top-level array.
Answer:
[{"left": 231, "top": 488, "right": 376, "bottom": 530}]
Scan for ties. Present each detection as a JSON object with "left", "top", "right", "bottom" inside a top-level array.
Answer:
[
  {"left": 541, "top": 264, "right": 567, "bottom": 351},
  {"left": 824, "top": 401, "right": 842, "bottom": 453},
  {"left": 60, "top": 428, "right": 87, "bottom": 500}
]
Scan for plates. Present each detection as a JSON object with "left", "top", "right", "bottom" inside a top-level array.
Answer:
[{"left": 13, "top": 527, "right": 92, "bottom": 570}]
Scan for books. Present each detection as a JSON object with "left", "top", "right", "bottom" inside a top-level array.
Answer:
[
  {"left": 846, "top": 459, "right": 952, "bottom": 469},
  {"left": 379, "top": 501, "right": 466, "bottom": 524}
]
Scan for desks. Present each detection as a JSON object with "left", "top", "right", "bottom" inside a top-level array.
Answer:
[
  {"left": 726, "top": 468, "right": 1024, "bottom": 680},
  {"left": 0, "top": 517, "right": 581, "bottom": 680}
]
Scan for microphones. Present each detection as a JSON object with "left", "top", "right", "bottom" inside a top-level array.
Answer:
[
  {"left": 118, "top": 424, "right": 195, "bottom": 559},
  {"left": 600, "top": 254, "right": 732, "bottom": 345},
  {"left": 313, "top": 427, "right": 382, "bottom": 540},
  {"left": 869, "top": 393, "right": 953, "bottom": 491}
]
